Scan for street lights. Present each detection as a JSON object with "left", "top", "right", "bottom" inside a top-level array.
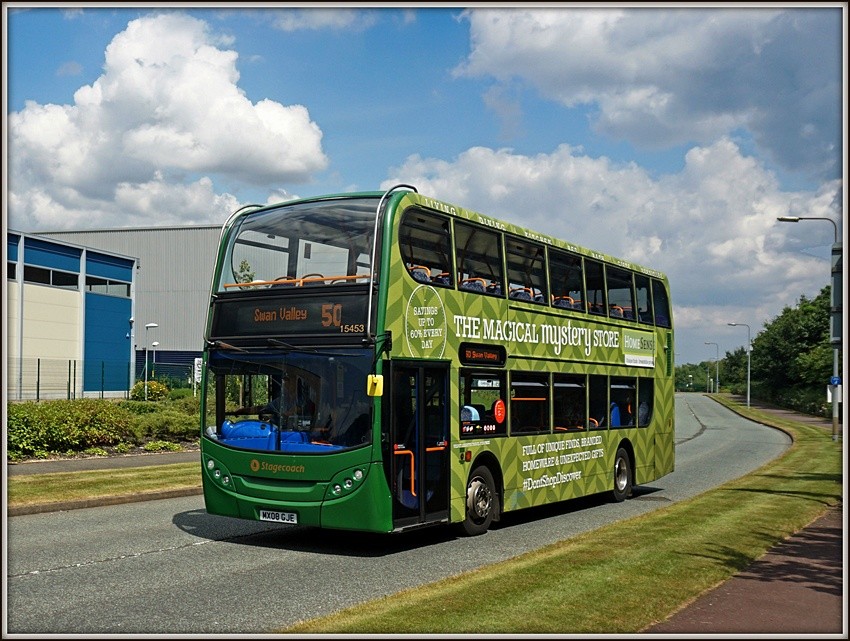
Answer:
[
  {"left": 704, "top": 343, "right": 719, "bottom": 392},
  {"left": 145, "top": 323, "right": 159, "bottom": 400},
  {"left": 727, "top": 323, "right": 751, "bottom": 409},
  {"left": 777, "top": 216, "right": 839, "bottom": 443}
]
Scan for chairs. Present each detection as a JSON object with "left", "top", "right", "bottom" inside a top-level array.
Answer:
[
  {"left": 408, "top": 266, "right": 642, "bottom": 323},
  {"left": 519, "top": 397, "right": 650, "bottom": 435},
  {"left": 269, "top": 272, "right": 354, "bottom": 288}
]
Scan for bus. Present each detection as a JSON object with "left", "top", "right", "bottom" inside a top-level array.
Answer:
[{"left": 200, "top": 183, "right": 677, "bottom": 538}]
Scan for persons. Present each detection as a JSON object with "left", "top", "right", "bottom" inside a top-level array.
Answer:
[{"left": 235, "top": 375, "right": 314, "bottom": 432}]
